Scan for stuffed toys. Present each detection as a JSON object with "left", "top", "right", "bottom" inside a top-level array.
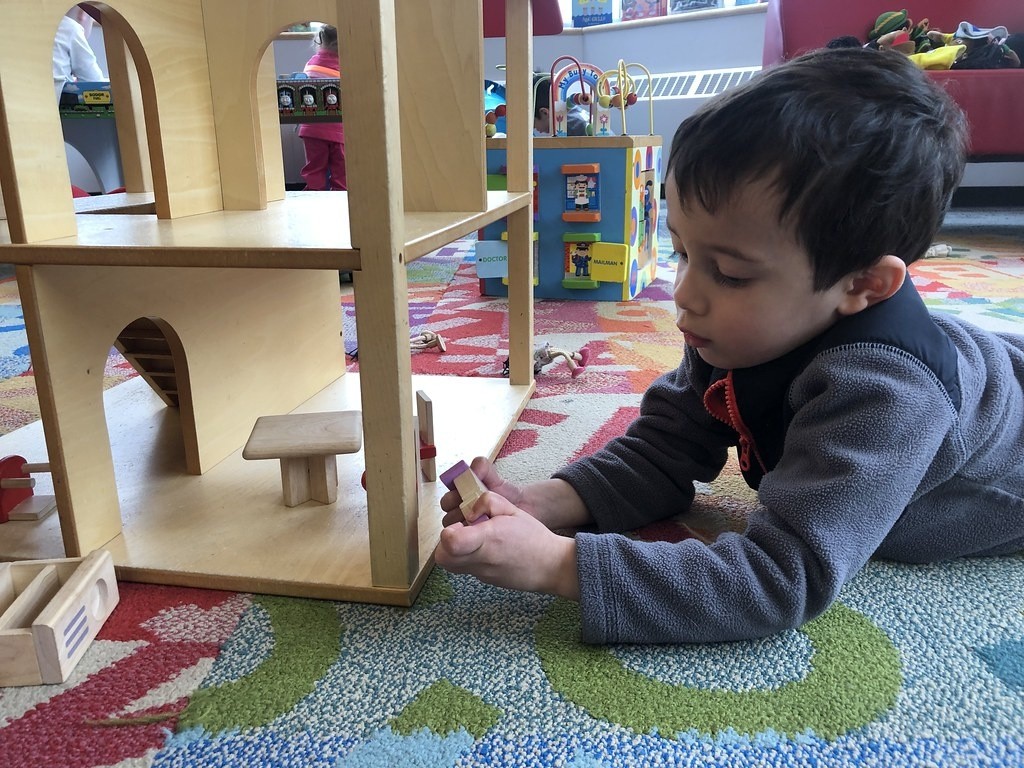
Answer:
[{"left": 826, "top": 11, "right": 1020, "bottom": 70}]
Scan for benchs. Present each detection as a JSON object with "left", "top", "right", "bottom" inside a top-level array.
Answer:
[{"left": 761, "top": 0, "right": 1024, "bottom": 213}]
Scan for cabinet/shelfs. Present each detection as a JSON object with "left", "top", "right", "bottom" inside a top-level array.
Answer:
[{"left": 0, "top": 0, "right": 535, "bottom": 609}]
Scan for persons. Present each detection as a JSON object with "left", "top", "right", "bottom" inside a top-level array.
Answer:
[
  {"left": 533, "top": 76, "right": 593, "bottom": 136},
  {"left": 503, "top": 345, "right": 588, "bottom": 379},
  {"left": 298, "top": 25, "right": 347, "bottom": 192},
  {"left": 53, "top": 5, "right": 105, "bottom": 107},
  {"left": 344, "top": 331, "right": 447, "bottom": 363},
  {"left": 435, "top": 47, "right": 1024, "bottom": 645}
]
